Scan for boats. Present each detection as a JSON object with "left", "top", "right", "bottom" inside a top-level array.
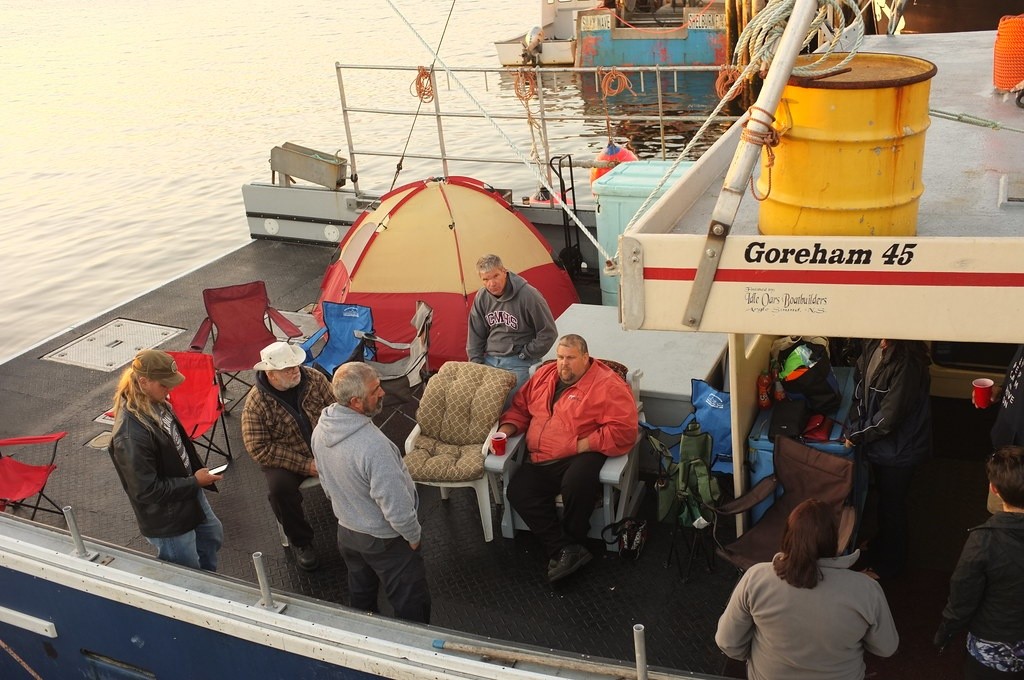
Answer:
[{"left": 0, "top": 0, "right": 1024, "bottom": 680}]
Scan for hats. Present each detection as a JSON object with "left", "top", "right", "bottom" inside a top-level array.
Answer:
[
  {"left": 132, "top": 349, "right": 185, "bottom": 387},
  {"left": 253, "top": 341, "right": 306, "bottom": 370}
]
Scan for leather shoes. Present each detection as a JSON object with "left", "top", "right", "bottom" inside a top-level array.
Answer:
[
  {"left": 296, "top": 542, "right": 318, "bottom": 570},
  {"left": 548, "top": 543, "right": 593, "bottom": 581}
]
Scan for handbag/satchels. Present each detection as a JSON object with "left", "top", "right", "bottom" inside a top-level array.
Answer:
[
  {"left": 767, "top": 333, "right": 849, "bottom": 445},
  {"left": 600, "top": 516, "right": 647, "bottom": 561}
]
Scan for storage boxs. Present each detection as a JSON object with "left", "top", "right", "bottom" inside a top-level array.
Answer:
[{"left": 925, "top": 341, "right": 1009, "bottom": 400}]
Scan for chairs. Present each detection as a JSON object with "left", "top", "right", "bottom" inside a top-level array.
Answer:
[
  {"left": 715, "top": 433, "right": 858, "bottom": 573},
  {"left": 647, "top": 422, "right": 730, "bottom": 581},
  {"left": 484, "top": 361, "right": 646, "bottom": 553},
  {"left": 636, "top": 378, "right": 732, "bottom": 523},
  {"left": 0, "top": 431, "right": 67, "bottom": 520},
  {"left": 399, "top": 360, "right": 516, "bottom": 542},
  {"left": 104, "top": 280, "right": 434, "bottom": 470}
]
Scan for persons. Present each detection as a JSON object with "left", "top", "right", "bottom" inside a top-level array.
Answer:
[
  {"left": 717, "top": 499, "right": 900, "bottom": 680},
  {"left": 241, "top": 340, "right": 338, "bottom": 569},
  {"left": 842, "top": 338, "right": 933, "bottom": 580},
  {"left": 972, "top": 344, "right": 1024, "bottom": 448},
  {"left": 311, "top": 361, "right": 431, "bottom": 624},
  {"left": 491, "top": 334, "right": 637, "bottom": 581},
  {"left": 109, "top": 350, "right": 223, "bottom": 572},
  {"left": 467, "top": 254, "right": 558, "bottom": 412},
  {"left": 933, "top": 446, "right": 1024, "bottom": 680}
]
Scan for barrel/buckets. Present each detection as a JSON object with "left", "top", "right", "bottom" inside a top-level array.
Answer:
[{"left": 757, "top": 51, "right": 938, "bottom": 236}]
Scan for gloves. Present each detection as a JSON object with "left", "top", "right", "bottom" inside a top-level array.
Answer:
[{"left": 933, "top": 624, "right": 955, "bottom": 650}]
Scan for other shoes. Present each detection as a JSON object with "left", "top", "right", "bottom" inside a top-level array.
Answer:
[
  {"left": 861, "top": 537, "right": 887, "bottom": 556},
  {"left": 879, "top": 561, "right": 899, "bottom": 580}
]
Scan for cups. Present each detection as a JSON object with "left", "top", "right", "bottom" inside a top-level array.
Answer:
[
  {"left": 972, "top": 378, "right": 994, "bottom": 409},
  {"left": 491, "top": 432, "right": 507, "bottom": 456}
]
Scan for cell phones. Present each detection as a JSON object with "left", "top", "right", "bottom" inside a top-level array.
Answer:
[{"left": 207, "top": 462, "right": 230, "bottom": 475}]
